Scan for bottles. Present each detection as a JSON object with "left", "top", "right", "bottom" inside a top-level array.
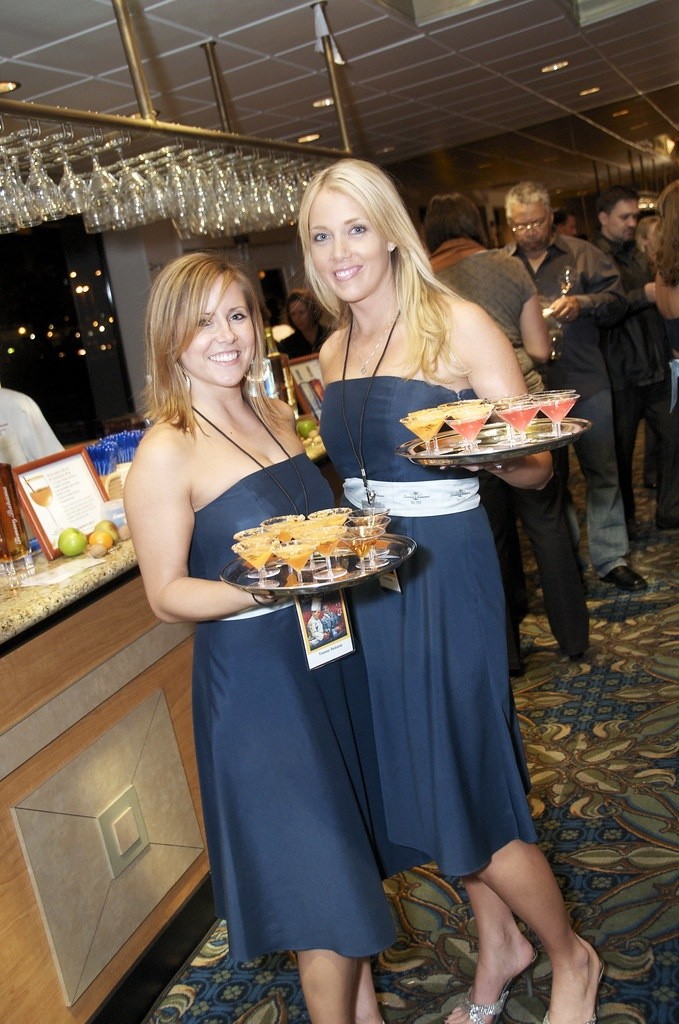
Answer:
[
  {"left": 295, "top": 366, "right": 325, "bottom": 421},
  {"left": 264, "top": 326, "right": 296, "bottom": 407}
]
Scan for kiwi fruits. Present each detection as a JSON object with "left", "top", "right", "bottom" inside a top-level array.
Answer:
[
  {"left": 118, "top": 525, "right": 131, "bottom": 540},
  {"left": 90, "top": 545, "right": 107, "bottom": 558}
]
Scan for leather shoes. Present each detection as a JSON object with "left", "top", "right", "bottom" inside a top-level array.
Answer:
[{"left": 600, "top": 565, "right": 647, "bottom": 593}]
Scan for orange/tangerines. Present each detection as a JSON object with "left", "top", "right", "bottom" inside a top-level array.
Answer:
[{"left": 89, "top": 532, "right": 113, "bottom": 549}]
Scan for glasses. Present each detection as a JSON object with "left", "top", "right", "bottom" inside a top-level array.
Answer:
[{"left": 511, "top": 215, "right": 548, "bottom": 232}]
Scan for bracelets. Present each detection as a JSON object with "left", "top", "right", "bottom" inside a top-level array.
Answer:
[{"left": 252, "top": 594, "right": 265, "bottom": 606}]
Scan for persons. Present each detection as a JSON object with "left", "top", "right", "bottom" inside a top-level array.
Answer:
[
  {"left": 0, "top": 387, "right": 66, "bottom": 468},
  {"left": 278, "top": 288, "right": 337, "bottom": 358},
  {"left": 306, "top": 597, "right": 343, "bottom": 648},
  {"left": 421, "top": 192, "right": 589, "bottom": 676},
  {"left": 123, "top": 251, "right": 399, "bottom": 1024},
  {"left": 298, "top": 159, "right": 606, "bottom": 1024},
  {"left": 502, "top": 179, "right": 679, "bottom": 591}
]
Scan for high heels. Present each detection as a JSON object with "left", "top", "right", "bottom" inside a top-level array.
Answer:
[
  {"left": 446, "top": 949, "right": 537, "bottom": 1023},
  {"left": 541, "top": 957, "right": 605, "bottom": 1024}
]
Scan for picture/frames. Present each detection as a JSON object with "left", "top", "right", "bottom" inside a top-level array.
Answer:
[
  {"left": 12, "top": 444, "right": 109, "bottom": 561},
  {"left": 288, "top": 353, "right": 324, "bottom": 426}
]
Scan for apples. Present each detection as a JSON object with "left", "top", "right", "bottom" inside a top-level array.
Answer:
[
  {"left": 94, "top": 521, "right": 119, "bottom": 542},
  {"left": 297, "top": 420, "right": 316, "bottom": 438},
  {"left": 58, "top": 528, "right": 88, "bottom": 557}
]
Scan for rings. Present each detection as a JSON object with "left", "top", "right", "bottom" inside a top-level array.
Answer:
[
  {"left": 494, "top": 463, "right": 502, "bottom": 470},
  {"left": 566, "top": 316, "right": 570, "bottom": 319}
]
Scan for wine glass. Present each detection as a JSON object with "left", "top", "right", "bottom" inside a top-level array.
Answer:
[
  {"left": 336, "top": 507, "right": 392, "bottom": 579},
  {"left": 230, "top": 507, "right": 352, "bottom": 579},
  {"left": 547, "top": 319, "right": 564, "bottom": 358},
  {"left": 488, "top": 389, "right": 580, "bottom": 446},
  {"left": 399, "top": 399, "right": 496, "bottom": 456},
  {"left": 0, "top": 128, "right": 327, "bottom": 240},
  {"left": 25, "top": 475, "right": 64, "bottom": 532}
]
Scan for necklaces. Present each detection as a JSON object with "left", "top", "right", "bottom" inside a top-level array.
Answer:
[{"left": 350, "top": 313, "right": 397, "bottom": 373}]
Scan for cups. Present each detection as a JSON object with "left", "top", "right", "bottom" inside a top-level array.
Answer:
[{"left": 0, "top": 463, "right": 34, "bottom": 576}]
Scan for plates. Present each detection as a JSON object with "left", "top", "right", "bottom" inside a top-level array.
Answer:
[{"left": 542, "top": 308, "right": 554, "bottom": 319}]
